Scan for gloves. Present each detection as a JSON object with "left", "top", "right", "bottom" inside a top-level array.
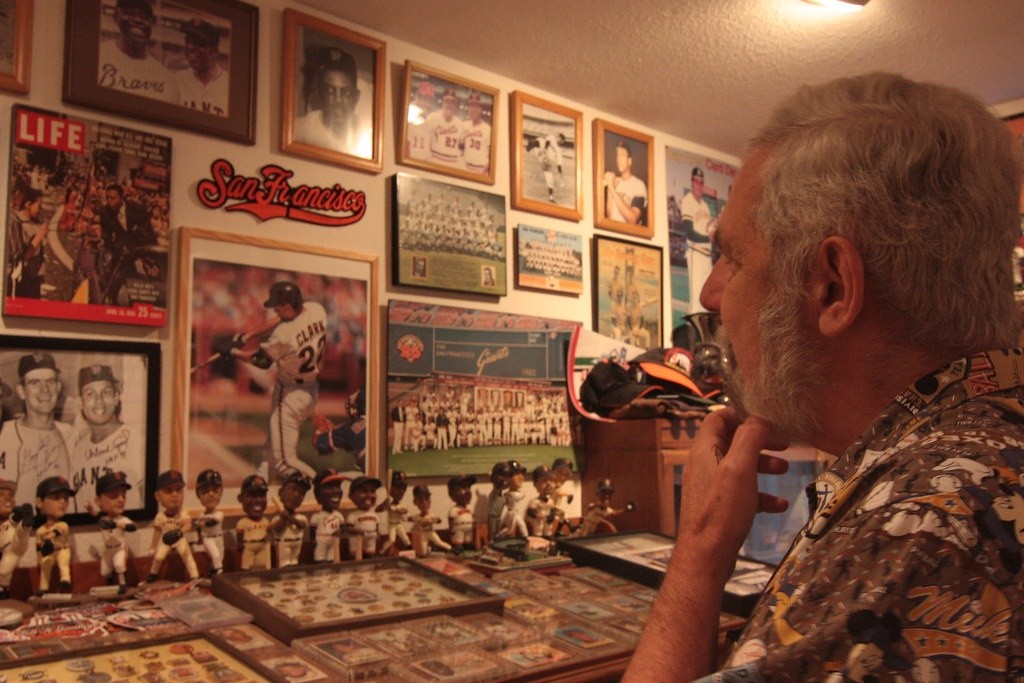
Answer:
[{"left": 215, "top": 333, "right": 249, "bottom": 361}]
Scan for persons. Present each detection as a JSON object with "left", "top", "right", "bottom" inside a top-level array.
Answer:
[
  {"left": 608, "top": 267, "right": 650, "bottom": 349},
  {"left": 173, "top": 18, "right": 228, "bottom": 118},
  {"left": 292, "top": 48, "right": 372, "bottom": 159},
  {"left": 564, "top": 628, "right": 613, "bottom": 648},
  {"left": 7, "top": 153, "right": 170, "bottom": 307},
  {"left": 406, "top": 81, "right": 491, "bottom": 175},
  {"left": 484, "top": 268, "right": 495, "bottom": 286},
  {"left": 604, "top": 141, "right": 647, "bottom": 225},
  {"left": 618, "top": 79, "right": 1024, "bottom": 683},
  {"left": 214, "top": 281, "right": 366, "bottom": 482},
  {"left": 390, "top": 386, "right": 571, "bottom": 454},
  {"left": 681, "top": 167, "right": 714, "bottom": 313},
  {"left": 401, "top": 191, "right": 505, "bottom": 262},
  {"left": 414, "top": 259, "right": 425, "bottom": 277},
  {"left": 526, "top": 133, "right": 565, "bottom": 203},
  {"left": 0, "top": 353, "right": 129, "bottom": 512},
  {"left": 518, "top": 238, "right": 582, "bottom": 289},
  {"left": 98, "top": 0, "right": 176, "bottom": 105},
  {"left": 0, "top": 456, "right": 639, "bottom": 598},
  {"left": 217, "top": 627, "right": 379, "bottom": 683}
]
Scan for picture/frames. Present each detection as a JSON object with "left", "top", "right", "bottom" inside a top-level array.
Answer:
[
  {"left": 397, "top": 59, "right": 500, "bottom": 186},
  {"left": 509, "top": 91, "right": 585, "bottom": 222},
  {"left": 1, "top": 336, "right": 159, "bottom": 523},
  {"left": 60, "top": 0, "right": 260, "bottom": 146},
  {"left": 279, "top": 7, "right": 386, "bottom": 175},
  {"left": 593, "top": 118, "right": 654, "bottom": 238},
  {"left": 172, "top": 227, "right": 381, "bottom": 521},
  {"left": 0, "top": 0, "right": 34, "bottom": 95},
  {"left": 593, "top": 234, "right": 664, "bottom": 350}
]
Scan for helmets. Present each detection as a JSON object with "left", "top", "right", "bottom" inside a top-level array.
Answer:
[{"left": 264, "top": 281, "right": 303, "bottom": 311}]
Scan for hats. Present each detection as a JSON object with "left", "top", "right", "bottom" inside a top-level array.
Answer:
[
  {"left": 19, "top": 353, "right": 61, "bottom": 380},
  {"left": 79, "top": 365, "right": 116, "bottom": 388},
  {"left": 318, "top": 49, "right": 356, "bottom": 82},
  {"left": 179, "top": 18, "right": 219, "bottom": 47},
  {"left": 693, "top": 167, "right": 704, "bottom": 180},
  {"left": 467, "top": 95, "right": 483, "bottom": 108},
  {"left": 118, "top": 0, "right": 153, "bottom": 17},
  {"left": 419, "top": 82, "right": 435, "bottom": 100},
  {"left": 441, "top": 87, "right": 458, "bottom": 101}
]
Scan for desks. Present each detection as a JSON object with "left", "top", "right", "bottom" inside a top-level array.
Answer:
[{"left": 576, "top": 414, "right": 705, "bottom": 541}]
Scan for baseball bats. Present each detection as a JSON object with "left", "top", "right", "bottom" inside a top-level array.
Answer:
[{"left": 191, "top": 351, "right": 220, "bottom": 375}]
